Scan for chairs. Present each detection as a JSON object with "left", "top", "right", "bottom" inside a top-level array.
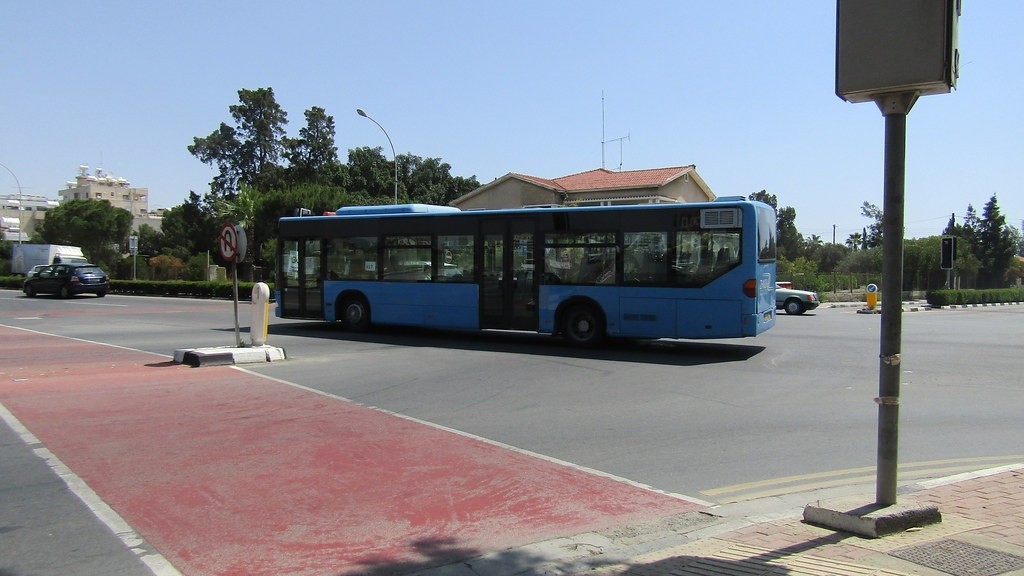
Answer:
[
  {"left": 695, "top": 250, "right": 715, "bottom": 274},
  {"left": 716, "top": 248, "right": 731, "bottom": 266}
]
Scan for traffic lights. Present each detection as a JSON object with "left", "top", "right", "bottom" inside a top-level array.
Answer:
[{"left": 941, "top": 237, "right": 953, "bottom": 269}]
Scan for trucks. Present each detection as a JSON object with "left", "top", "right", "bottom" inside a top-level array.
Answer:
[{"left": 12, "top": 243, "right": 89, "bottom": 273}]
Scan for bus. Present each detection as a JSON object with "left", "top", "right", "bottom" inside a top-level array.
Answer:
[{"left": 273, "top": 194, "right": 776, "bottom": 350}]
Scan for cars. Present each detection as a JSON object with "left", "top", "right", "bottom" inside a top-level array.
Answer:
[
  {"left": 774, "top": 283, "right": 820, "bottom": 315},
  {"left": 23, "top": 262, "right": 111, "bottom": 299},
  {"left": 27, "top": 265, "right": 59, "bottom": 278}
]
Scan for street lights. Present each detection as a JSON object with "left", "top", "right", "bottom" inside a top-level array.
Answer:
[{"left": 356, "top": 108, "right": 398, "bottom": 204}]
